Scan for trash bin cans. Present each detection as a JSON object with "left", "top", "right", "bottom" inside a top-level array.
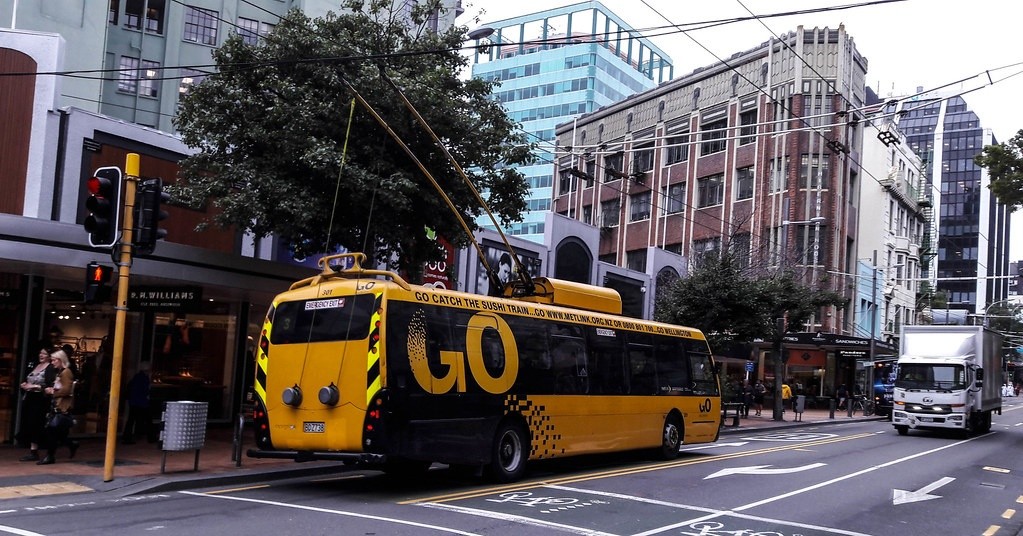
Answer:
[
  {"left": 793, "top": 395, "right": 806, "bottom": 413},
  {"left": 159, "top": 400, "right": 208, "bottom": 452}
]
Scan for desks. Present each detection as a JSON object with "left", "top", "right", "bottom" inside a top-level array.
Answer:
[{"left": 164, "top": 375, "right": 204, "bottom": 383}]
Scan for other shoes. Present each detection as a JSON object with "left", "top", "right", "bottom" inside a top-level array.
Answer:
[
  {"left": 67, "top": 440, "right": 80, "bottom": 459},
  {"left": 755, "top": 412, "right": 761, "bottom": 416},
  {"left": 19, "top": 449, "right": 39, "bottom": 462},
  {"left": 35, "top": 452, "right": 57, "bottom": 465}
]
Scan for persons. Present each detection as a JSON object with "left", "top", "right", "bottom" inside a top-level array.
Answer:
[
  {"left": 782, "top": 380, "right": 794, "bottom": 413},
  {"left": 243, "top": 335, "right": 256, "bottom": 405},
  {"left": 904, "top": 365, "right": 925, "bottom": 382},
  {"left": 752, "top": 379, "right": 767, "bottom": 417},
  {"left": 837, "top": 381, "right": 850, "bottom": 411},
  {"left": 739, "top": 379, "right": 752, "bottom": 419},
  {"left": 15, "top": 344, "right": 78, "bottom": 466},
  {"left": 120, "top": 361, "right": 154, "bottom": 446},
  {"left": 487, "top": 253, "right": 512, "bottom": 297}
]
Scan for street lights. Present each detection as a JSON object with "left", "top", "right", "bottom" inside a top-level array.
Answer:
[
  {"left": 984, "top": 298, "right": 1015, "bottom": 327},
  {"left": 870, "top": 264, "right": 904, "bottom": 402},
  {"left": 946, "top": 300, "right": 970, "bottom": 325}
]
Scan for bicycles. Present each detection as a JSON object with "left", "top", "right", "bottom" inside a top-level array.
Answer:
[{"left": 852, "top": 393, "right": 875, "bottom": 415}]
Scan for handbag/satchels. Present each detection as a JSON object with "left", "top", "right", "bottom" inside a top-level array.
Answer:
[{"left": 52, "top": 377, "right": 77, "bottom": 397}]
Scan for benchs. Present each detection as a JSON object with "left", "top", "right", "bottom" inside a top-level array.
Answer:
[{"left": 721, "top": 402, "right": 744, "bottom": 426}]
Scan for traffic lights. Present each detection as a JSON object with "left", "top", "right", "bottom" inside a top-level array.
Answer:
[
  {"left": 87, "top": 261, "right": 114, "bottom": 285},
  {"left": 84, "top": 166, "right": 122, "bottom": 247}
]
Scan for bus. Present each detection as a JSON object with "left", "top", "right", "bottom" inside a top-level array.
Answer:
[{"left": 246, "top": 252, "right": 722, "bottom": 481}]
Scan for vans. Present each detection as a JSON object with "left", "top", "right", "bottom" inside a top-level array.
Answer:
[{"left": 874, "top": 358, "right": 897, "bottom": 415}]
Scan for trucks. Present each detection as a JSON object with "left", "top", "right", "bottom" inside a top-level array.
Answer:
[{"left": 892, "top": 325, "right": 1003, "bottom": 434}]
